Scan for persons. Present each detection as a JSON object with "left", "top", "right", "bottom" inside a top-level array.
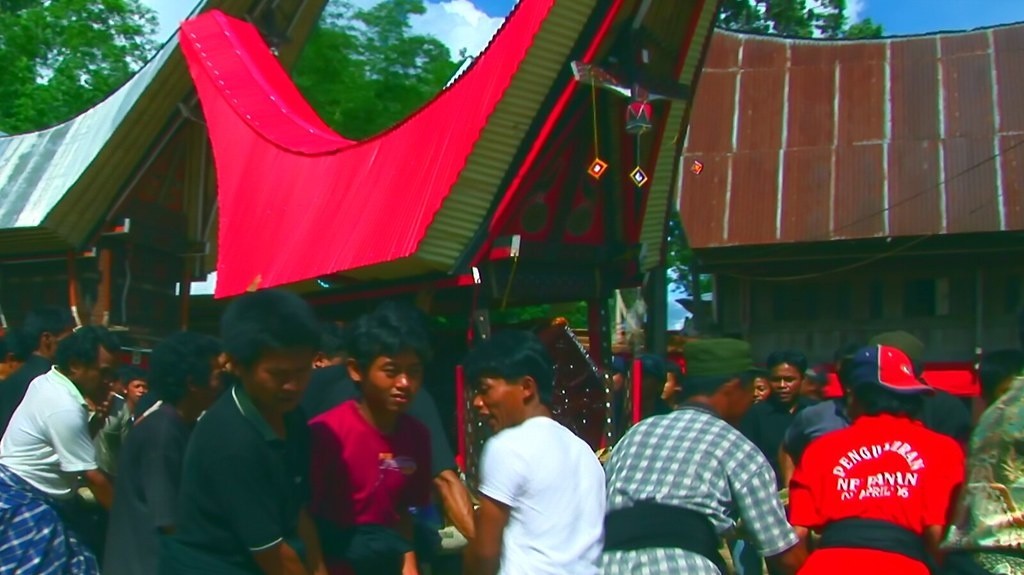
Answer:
[
  {"left": 1, "top": 283, "right": 472, "bottom": 574},
  {"left": 590, "top": 319, "right": 1024, "bottom": 526},
  {"left": 461, "top": 326, "right": 607, "bottom": 574},
  {"left": 787, "top": 345, "right": 966, "bottom": 575},
  {"left": 938, "top": 368, "right": 1023, "bottom": 575},
  {"left": 602, "top": 337, "right": 808, "bottom": 574}
]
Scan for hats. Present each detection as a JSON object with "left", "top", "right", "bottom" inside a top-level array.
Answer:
[
  {"left": 684, "top": 337, "right": 771, "bottom": 375},
  {"left": 844, "top": 344, "right": 935, "bottom": 395}
]
